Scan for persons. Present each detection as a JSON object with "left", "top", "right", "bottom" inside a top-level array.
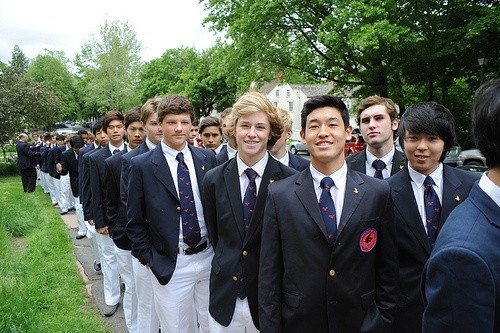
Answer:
[
  {"left": 16, "top": 133, "right": 41, "bottom": 193},
  {"left": 32, "top": 93, "right": 309, "bottom": 332},
  {"left": 201, "top": 93, "right": 301, "bottom": 333},
  {"left": 421, "top": 78, "right": 500, "bottom": 333},
  {"left": 345, "top": 96, "right": 406, "bottom": 180},
  {"left": 384, "top": 101, "right": 483, "bottom": 333},
  {"left": 259, "top": 94, "right": 392, "bottom": 333}
]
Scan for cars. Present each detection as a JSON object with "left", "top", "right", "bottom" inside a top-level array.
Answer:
[
  {"left": 393, "top": 135, "right": 489, "bottom": 172},
  {"left": 289, "top": 139, "right": 308, "bottom": 155},
  {"left": 344, "top": 127, "right": 367, "bottom": 158},
  {"left": 32, "top": 121, "right": 91, "bottom": 138}
]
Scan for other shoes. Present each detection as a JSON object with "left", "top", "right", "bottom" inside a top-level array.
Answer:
[
  {"left": 93, "top": 261, "right": 101, "bottom": 271},
  {"left": 76, "top": 235, "right": 86, "bottom": 239},
  {"left": 61, "top": 212, "right": 67, "bottom": 215},
  {"left": 68, "top": 207, "right": 73, "bottom": 212},
  {"left": 104, "top": 303, "right": 118, "bottom": 316}
]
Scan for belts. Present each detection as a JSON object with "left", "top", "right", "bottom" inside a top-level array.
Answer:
[{"left": 177, "top": 241, "right": 207, "bottom": 254}]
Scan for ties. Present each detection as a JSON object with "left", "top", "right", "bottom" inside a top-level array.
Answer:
[
  {"left": 176, "top": 152, "right": 201, "bottom": 250},
  {"left": 113, "top": 149, "right": 121, "bottom": 154},
  {"left": 77, "top": 153, "right": 79, "bottom": 159},
  {"left": 318, "top": 177, "right": 337, "bottom": 241},
  {"left": 423, "top": 177, "right": 442, "bottom": 243},
  {"left": 242, "top": 168, "right": 258, "bottom": 235},
  {"left": 372, "top": 160, "right": 387, "bottom": 179}
]
